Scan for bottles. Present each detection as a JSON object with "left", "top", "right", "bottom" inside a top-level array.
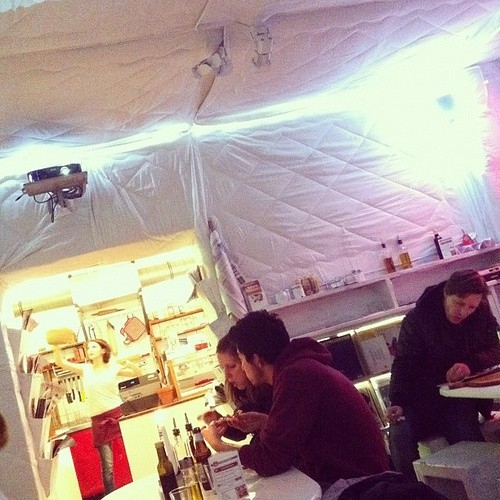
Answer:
[
  {"left": 185, "top": 424, "right": 195, "bottom": 458},
  {"left": 434, "top": 229, "right": 444, "bottom": 260},
  {"left": 154, "top": 442, "right": 181, "bottom": 500},
  {"left": 173, "top": 429, "right": 195, "bottom": 472},
  {"left": 194, "top": 428, "right": 212, "bottom": 490},
  {"left": 381, "top": 244, "right": 396, "bottom": 273},
  {"left": 397, "top": 240, "right": 413, "bottom": 268}
]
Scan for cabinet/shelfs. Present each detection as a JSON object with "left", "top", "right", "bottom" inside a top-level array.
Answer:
[
  {"left": 264, "top": 244, "right": 500, "bottom": 472},
  {"left": 36, "top": 287, "right": 220, "bottom": 400}
]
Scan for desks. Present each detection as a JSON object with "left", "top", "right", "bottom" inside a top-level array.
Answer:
[
  {"left": 100, "top": 463, "right": 322, "bottom": 500},
  {"left": 439, "top": 383, "right": 500, "bottom": 399}
]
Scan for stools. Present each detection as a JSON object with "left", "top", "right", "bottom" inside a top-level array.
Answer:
[
  {"left": 412, "top": 440, "right": 500, "bottom": 500},
  {"left": 418, "top": 434, "right": 450, "bottom": 458}
]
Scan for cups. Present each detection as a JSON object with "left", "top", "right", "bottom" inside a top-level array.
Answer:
[
  {"left": 181, "top": 466, "right": 204, "bottom": 500},
  {"left": 169, "top": 486, "right": 194, "bottom": 500}
]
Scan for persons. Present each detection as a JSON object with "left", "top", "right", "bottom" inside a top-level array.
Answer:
[
  {"left": 200, "top": 308, "right": 390, "bottom": 495},
  {"left": 52, "top": 337, "right": 141, "bottom": 496},
  {"left": 386, "top": 269, "right": 500, "bottom": 483}
]
